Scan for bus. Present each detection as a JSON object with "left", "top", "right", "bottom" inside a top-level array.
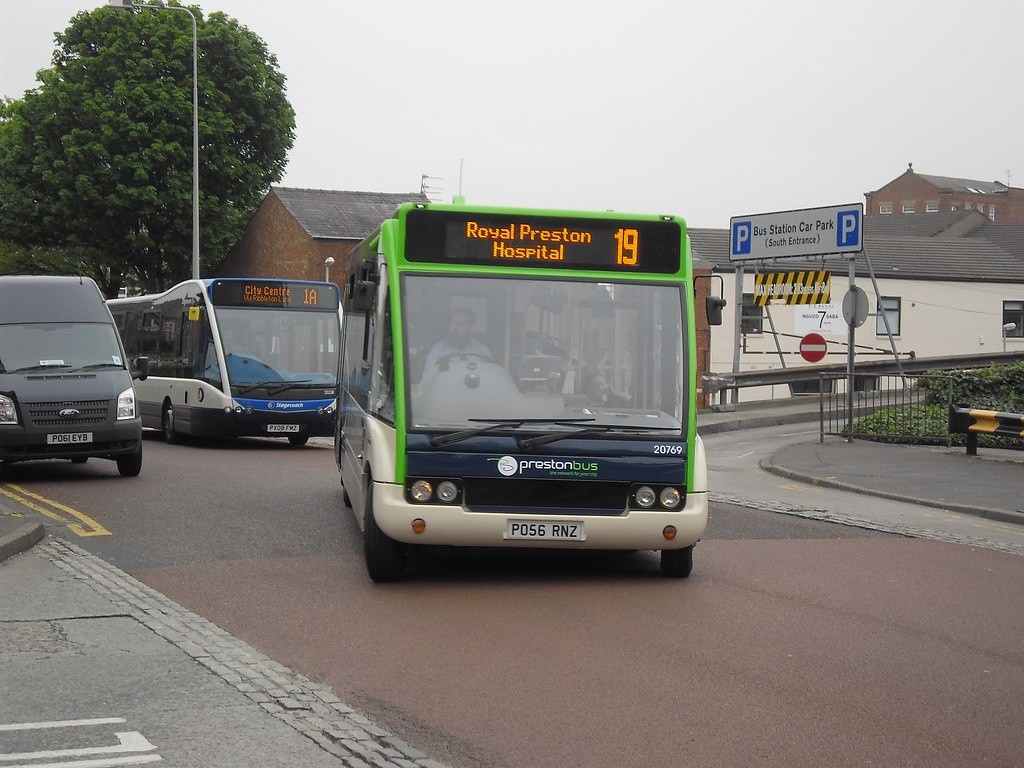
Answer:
[
  {"left": 104, "top": 277, "right": 343, "bottom": 447},
  {"left": 340, "top": 202, "right": 729, "bottom": 584}
]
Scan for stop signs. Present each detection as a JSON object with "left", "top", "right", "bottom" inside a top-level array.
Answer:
[{"left": 798, "top": 332, "right": 828, "bottom": 364}]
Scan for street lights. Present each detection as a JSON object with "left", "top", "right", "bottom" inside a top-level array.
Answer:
[
  {"left": 107, "top": 0, "right": 200, "bottom": 277},
  {"left": 1002, "top": 322, "right": 1017, "bottom": 352},
  {"left": 325, "top": 256, "right": 335, "bottom": 285}
]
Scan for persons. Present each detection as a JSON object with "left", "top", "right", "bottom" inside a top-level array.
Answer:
[
  {"left": 421, "top": 306, "right": 492, "bottom": 380},
  {"left": 582, "top": 372, "right": 632, "bottom": 408}
]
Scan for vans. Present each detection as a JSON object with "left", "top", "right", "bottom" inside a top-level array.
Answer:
[{"left": 0, "top": 272, "right": 148, "bottom": 479}]
